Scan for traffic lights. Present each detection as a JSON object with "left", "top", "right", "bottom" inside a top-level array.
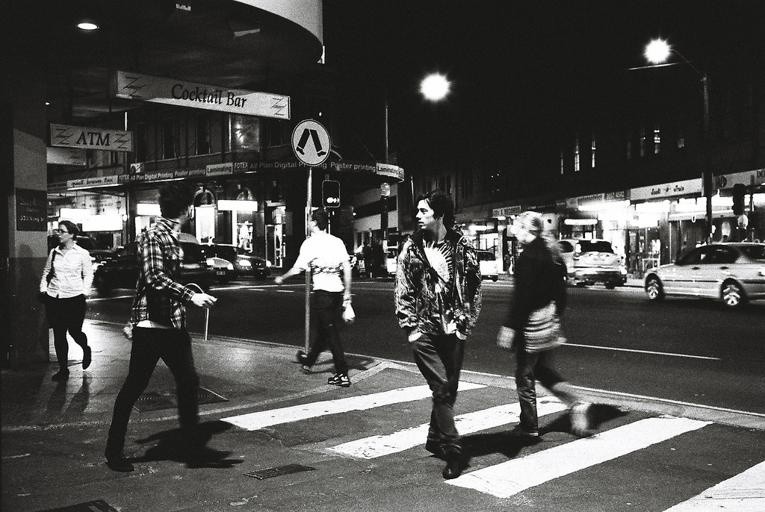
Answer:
[
  {"left": 321, "top": 180, "right": 341, "bottom": 208},
  {"left": 729, "top": 183, "right": 746, "bottom": 217}
]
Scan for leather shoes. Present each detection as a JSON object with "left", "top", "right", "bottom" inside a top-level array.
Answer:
[
  {"left": 425, "top": 436, "right": 453, "bottom": 460},
  {"left": 183, "top": 444, "right": 234, "bottom": 468},
  {"left": 103, "top": 446, "right": 135, "bottom": 474},
  {"left": 441, "top": 440, "right": 473, "bottom": 480}
]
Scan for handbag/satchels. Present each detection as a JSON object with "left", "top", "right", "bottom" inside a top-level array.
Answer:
[
  {"left": 46, "top": 250, "right": 57, "bottom": 283},
  {"left": 123, "top": 317, "right": 134, "bottom": 340}
]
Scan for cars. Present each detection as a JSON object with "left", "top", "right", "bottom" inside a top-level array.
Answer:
[
  {"left": 639, "top": 238, "right": 765, "bottom": 312},
  {"left": 45, "top": 227, "right": 270, "bottom": 288},
  {"left": 468, "top": 248, "right": 499, "bottom": 282}
]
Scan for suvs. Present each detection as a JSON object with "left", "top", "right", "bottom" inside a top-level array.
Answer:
[{"left": 552, "top": 236, "right": 630, "bottom": 292}]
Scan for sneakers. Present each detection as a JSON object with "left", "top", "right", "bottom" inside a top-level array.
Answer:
[
  {"left": 82, "top": 346, "right": 92, "bottom": 370},
  {"left": 501, "top": 429, "right": 541, "bottom": 445},
  {"left": 295, "top": 348, "right": 313, "bottom": 374},
  {"left": 327, "top": 371, "right": 352, "bottom": 388},
  {"left": 566, "top": 400, "right": 593, "bottom": 434},
  {"left": 51, "top": 369, "right": 70, "bottom": 382}
]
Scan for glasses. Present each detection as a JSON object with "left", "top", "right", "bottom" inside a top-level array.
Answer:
[{"left": 58, "top": 229, "right": 68, "bottom": 234}]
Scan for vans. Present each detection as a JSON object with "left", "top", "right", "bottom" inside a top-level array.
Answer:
[{"left": 357, "top": 245, "right": 398, "bottom": 275}]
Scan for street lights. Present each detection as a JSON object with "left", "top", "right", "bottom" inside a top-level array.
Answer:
[
  {"left": 419, "top": 70, "right": 454, "bottom": 210},
  {"left": 642, "top": 37, "right": 714, "bottom": 245}
]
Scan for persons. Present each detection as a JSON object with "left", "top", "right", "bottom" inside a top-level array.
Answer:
[
  {"left": 39, "top": 221, "right": 95, "bottom": 380},
  {"left": 395, "top": 189, "right": 481, "bottom": 479},
  {"left": 239, "top": 219, "right": 249, "bottom": 247},
  {"left": 362, "top": 238, "right": 385, "bottom": 277},
  {"left": 275, "top": 209, "right": 353, "bottom": 384},
  {"left": 105, "top": 183, "right": 229, "bottom": 471},
  {"left": 629, "top": 253, "right": 642, "bottom": 279},
  {"left": 496, "top": 210, "right": 590, "bottom": 442},
  {"left": 504, "top": 249, "right": 515, "bottom": 277}
]
